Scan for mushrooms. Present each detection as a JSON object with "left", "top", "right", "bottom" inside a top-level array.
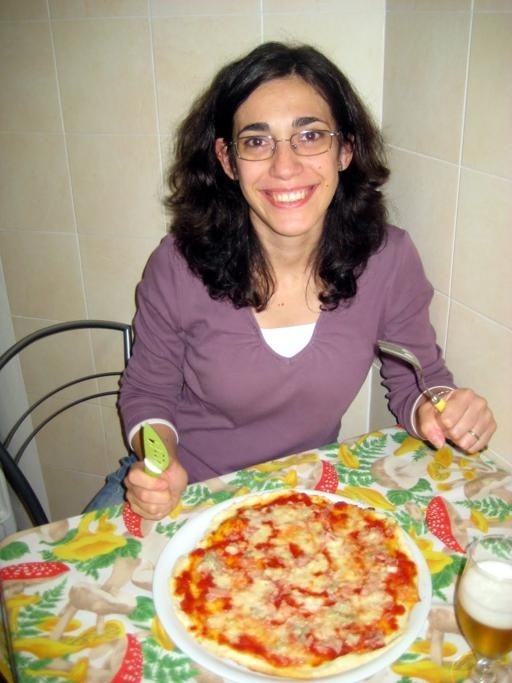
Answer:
[{"left": 1, "top": 431, "right": 511, "bottom": 682}]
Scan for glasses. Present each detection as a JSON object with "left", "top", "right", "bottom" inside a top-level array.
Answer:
[{"left": 229, "top": 129, "right": 341, "bottom": 161}]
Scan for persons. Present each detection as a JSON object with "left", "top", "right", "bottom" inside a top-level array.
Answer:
[{"left": 80, "top": 35, "right": 497, "bottom": 521}]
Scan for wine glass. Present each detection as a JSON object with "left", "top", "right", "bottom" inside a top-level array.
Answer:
[{"left": 452, "top": 532, "right": 512, "bottom": 682}]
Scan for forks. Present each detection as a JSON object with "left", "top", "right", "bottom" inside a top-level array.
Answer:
[{"left": 375, "top": 338, "right": 448, "bottom": 414}]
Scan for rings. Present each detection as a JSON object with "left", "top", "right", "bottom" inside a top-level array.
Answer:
[{"left": 468, "top": 429, "right": 480, "bottom": 441}]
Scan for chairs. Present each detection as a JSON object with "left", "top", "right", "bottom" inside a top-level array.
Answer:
[{"left": 0, "top": 317, "right": 135, "bottom": 527}]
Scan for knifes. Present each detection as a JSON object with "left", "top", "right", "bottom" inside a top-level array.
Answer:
[{"left": 142, "top": 419, "right": 170, "bottom": 478}]
